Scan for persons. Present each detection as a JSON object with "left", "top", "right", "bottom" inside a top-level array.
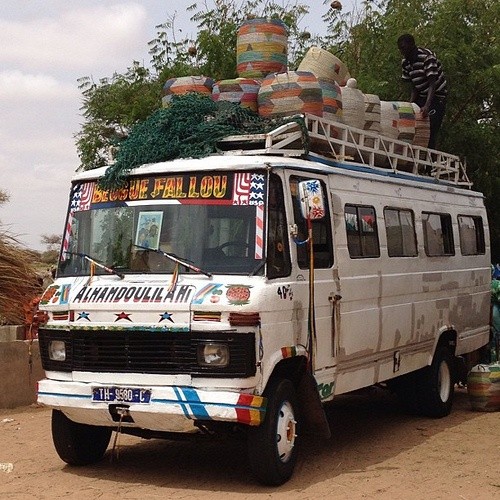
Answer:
[
  {"left": 146, "top": 225, "right": 158, "bottom": 249},
  {"left": 394, "top": 32, "right": 448, "bottom": 174},
  {"left": 138, "top": 228, "right": 147, "bottom": 249}
]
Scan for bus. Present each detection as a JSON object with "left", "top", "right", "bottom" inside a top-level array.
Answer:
[{"left": 27, "top": 111, "right": 492, "bottom": 486}]
